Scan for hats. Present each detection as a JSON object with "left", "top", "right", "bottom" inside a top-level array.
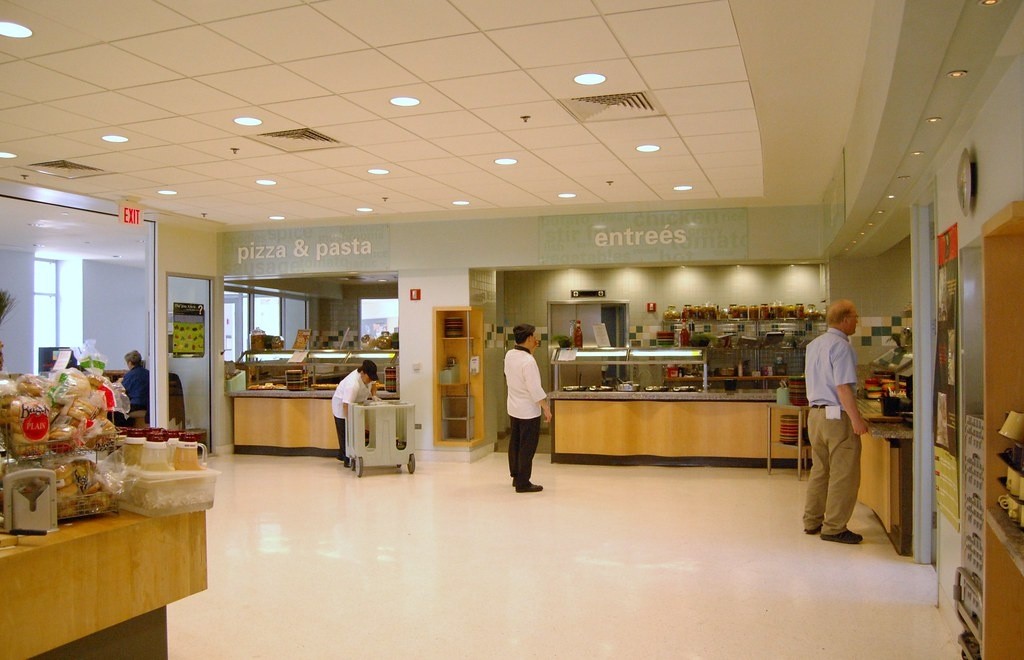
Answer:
[
  {"left": 513, "top": 324, "right": 535, "bottom": 338},
  {"left": 363, "top": 360, "right": 379, "bottom": 381}
]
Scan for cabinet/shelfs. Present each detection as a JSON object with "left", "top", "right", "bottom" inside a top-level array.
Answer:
[
  {"left": 433, "top": 306, "right": 484, "bottom": 447},
  {"left": 661, "top": 316, "right": 828, "bottom": 389},
  {"left": 766, "top": 404, "right": 808, "bottom": 480}
]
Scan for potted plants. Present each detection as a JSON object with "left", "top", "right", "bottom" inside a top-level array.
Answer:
[{"left": 0, "top": 291, "right": 19, "bottom": 371}]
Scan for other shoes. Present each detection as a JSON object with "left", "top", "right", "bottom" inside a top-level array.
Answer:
[
  {"left": 513, "top": 479, "right": 517, "bottom": 486},
  {"left": 820, "top": 530, "right": 863, "bottom": 544},
  {"left": 337, "top": 455, "right": 343, "bottom": 461},
  {"left": 515, "top": 482, "right": 543, "bottom": 492},
  {"left": 806, "top": 524, "right": 823, "bottom": 534},
  {"left": 344, "top": 462, "right": 350, "bottom": 467}
]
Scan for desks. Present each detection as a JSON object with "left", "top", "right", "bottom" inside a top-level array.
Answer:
[{"left": 0, "top": 508, "right": 208, "bottom": 660}]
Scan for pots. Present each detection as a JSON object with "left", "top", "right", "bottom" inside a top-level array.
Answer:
[
  {"left": 563, "top": 386, "right": 588, "bottom": 391},
  {"left": 589, "top": 385, "right": 613, "bottom": 391},
  {"left": 615, "top": 381, "right": 640, "bottom": 391}
]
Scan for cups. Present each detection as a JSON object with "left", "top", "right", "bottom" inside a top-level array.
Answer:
[{"left": 997, "top": 410, "right": 1024, "bottom": 529}]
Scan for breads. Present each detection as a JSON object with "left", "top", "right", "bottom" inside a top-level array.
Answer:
[
  {"left": 310, "top": 383, "right": 338, "bottom": 388},
  {"left": 0, "top": 371, "right": 114, "bottom": 518},
  {"left": 248, "top": 383, "right": 286, "bottom": 389},
  {"left": 375, "top": 382, "right": 384, "bottom": 387}
]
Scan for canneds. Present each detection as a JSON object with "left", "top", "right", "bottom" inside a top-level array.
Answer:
[{"left": 684, "top": 304, "right": 805, "bottom": 318}]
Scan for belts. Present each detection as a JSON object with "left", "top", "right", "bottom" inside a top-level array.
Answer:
[{"left": 812, "top": 405, "right": 828, "bottom": 408}]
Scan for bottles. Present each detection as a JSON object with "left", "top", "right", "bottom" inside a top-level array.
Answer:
[
  {"left": 737, "top": 358, "right": 753, "bottom": 377},
  {"left": 682, "top": 304, "right": 816, "bottom": 320},
  {"left": 569, "top": 320, "right": 583, "bottom": 351},
  {"left": 680, "top": 318, "right": 696, "bottom": 347},
  {"left": 777, "top": 380, "right": 789, "bottom": 405},
  {"left": 123, "top": 436, "right": 207, "bottom": 474}
]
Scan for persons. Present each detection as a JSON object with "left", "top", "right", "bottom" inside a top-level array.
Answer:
[
  {"left": 117, "top": 351, "right": 150, "bottom": 426},
  {"left": 504, "top": 324, "right": 552, "bottom": 493},
  {"left": 803, "top": 300, "right": 870, "bottom": 543},
  {"left": 332, "top": 359, "right": 379, "bottom": 467}
]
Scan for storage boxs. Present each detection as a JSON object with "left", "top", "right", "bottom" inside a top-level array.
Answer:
[{"left": 100, "top": 461, "right": 219, "bottom": 517}]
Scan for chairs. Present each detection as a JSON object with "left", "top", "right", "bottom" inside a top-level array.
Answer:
[{"left": 129, "top": 373, "right": 185, "bottom": 429}]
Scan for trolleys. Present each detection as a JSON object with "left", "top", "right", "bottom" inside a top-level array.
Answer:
[{"left": 344, "top": 399, "right": 417, "bottom": 478}]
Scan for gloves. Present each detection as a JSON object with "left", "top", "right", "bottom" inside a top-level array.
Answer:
[{"left": 372, "top": 396, "right": 381, "bottom": 401}]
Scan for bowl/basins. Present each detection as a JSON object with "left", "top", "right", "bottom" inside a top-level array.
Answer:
[
  {"left": 656, "top": 331, "right": 675, "bottom": 349},
  {"left": 788, "top": 377, "right": 808, "bottom": 406},
  {"left": 385, "top": 366, "right": 396, "bottom": 392},
  {"left": 865, "top": 374, "right": 895, "bottom": 398},
  {"left": 444, "top": 316, "right": 463, "bottom": 337},
  {"left": 779, "top": 414, "right": 798, "bottom": 444}
]
